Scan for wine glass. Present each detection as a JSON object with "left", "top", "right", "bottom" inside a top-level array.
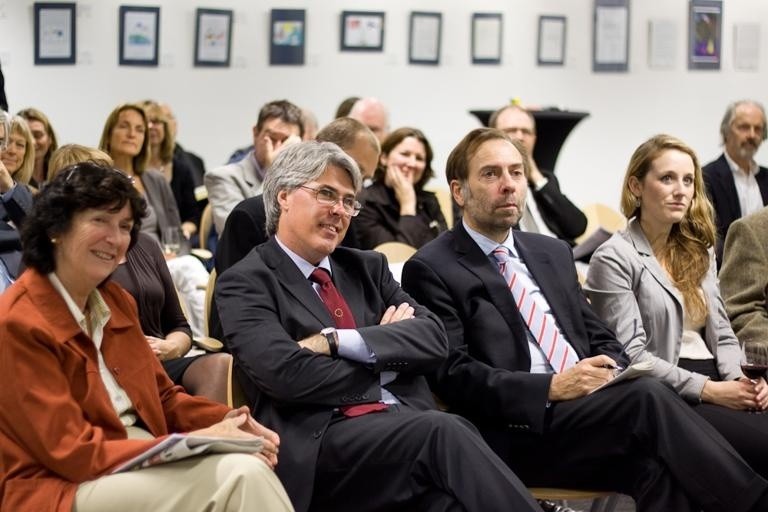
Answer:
[{"left": 738, "top": 339, "right": 768, "bottom": 388}]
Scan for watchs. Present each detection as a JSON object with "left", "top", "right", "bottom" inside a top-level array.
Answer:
[{"left": 317, "top": 323, "right": 340, "bottom": 360}]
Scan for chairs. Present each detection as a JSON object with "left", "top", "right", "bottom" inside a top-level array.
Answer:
[
  {"left": 524, "top": 483, "right": 622, "bottom": 512},
  {"left": 198, "top": 183, "right": 642, "bottom": 410}
]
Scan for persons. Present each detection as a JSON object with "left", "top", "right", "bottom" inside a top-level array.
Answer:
[
  {"left": 213, "top": 138, "right": 639, "bottom": 512},
  {"left": 594, "top": 130, "right": 766, "bottom": 458},
  {"left": 0, "top": 86, "right": 597, "bottom": 406},
  {"left": 703, "top": 95, "right": 764, "bottom": 275},
  {"left": 715, "top": 211, "right": 764, "bottom": 373},
  {"left": 0, "top": 161, "right": 297, "bottom": 512},
  {"left": 401, "top": 126, "right": 765, "bottom": 510}
]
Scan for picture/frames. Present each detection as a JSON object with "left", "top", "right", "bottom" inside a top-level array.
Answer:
[
  {"left": 31, "top": 0, "right": 78, "bottom": 68},
  {"left": 116, "top": 2, "right": 161, "bottom": 69},
  {"left": 686, "top": 0, "right": 724, "bottom": 71},
  {"left": 269, "top": 7, "right": 308, "bottom": 66},
  {"left": 469, "top": 10, "right": 505, "bottom": 65},
  {"left": 192, "top": 5, "right": 234, "bottom": 69},
  {"left": 536, "top": 13, "right": 569, "bottom": 67},
  {"left": 646, "top": 14, "right": 681, "bottom": 72},
  {"left": 591, "top": 1, "right": 631, "bottom": 74},
  {"left": 337, "top": 9, "right": 387, "bottom": 53},
  {"left": 408, "top": 9, "right": 443, "bottom": 67}
]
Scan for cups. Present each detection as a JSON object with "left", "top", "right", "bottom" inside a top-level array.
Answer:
[{"left": 159, "top": 226, "right": 182, "bottom": 255}]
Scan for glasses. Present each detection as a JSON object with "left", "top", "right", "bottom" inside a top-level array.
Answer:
[
  {"left": 0, "top": 140, "right": 10, "bottom": 153},
  {"left": 296, "top": 185, "right": 364, "bottom": 218},
  {"left": 67, "top": 162, "right": 135, "bottom": 186},
  {"left": 358, "top": 165, "right": 374, "bottom": 189}
]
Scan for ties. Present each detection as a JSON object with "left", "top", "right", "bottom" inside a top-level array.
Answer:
[
  {"left": 492, "top": 246, "right": 575, "bottom": 374},
  {"left": 311, "top": 267, "right": 390, "bottom": 416}
]
